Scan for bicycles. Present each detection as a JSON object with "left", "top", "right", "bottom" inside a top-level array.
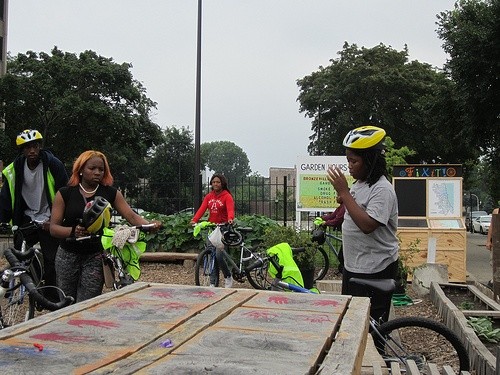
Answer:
[
  {"left": 0, "top": 246, "right": 74, "bottom": 311},
  {"left": 295, "top": 218, "right": 342, "bottom": 285},
  {"left": 0, "top": 220, "right": 48, "bottom": 327},
  {"left": 245, "top": 247, "right": 470, "bottom": 375},
  {"left": 193, "top": 222, "right": 271, "bottom": 290},
  {"left": 64, "top": 222, "right": 158, "bottom": 289}
]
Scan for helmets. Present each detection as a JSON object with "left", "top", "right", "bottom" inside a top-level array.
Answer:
[
  {"left": 79, "top": 196, "right": 114, "bottom": 234},
  {"left": 16, "top": 127, "right": 44, "bottom": 147},
  {"left": 342, "top": 125, "right": 387, "bottom": 153},
  {"left": 220, "top": 225, "right": 243, "bottom": 247}
]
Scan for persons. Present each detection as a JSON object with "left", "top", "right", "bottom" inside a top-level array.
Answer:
[
  {"left": 486, "top": 199, "right": 500, "bottom": 289},
  {"left": 318, "top": 124, "right": 401, "bottom": 372},
  {"left": 312, "top": 191, "right": 344, "bottom": 268},
  {"left": 48, "top": 151, "right": 163, "bottom": 304},
  {"left": 189, "top": 173, "right": 236, "bottom": 289},
  {"left": 0, "top": 159, "right": 12, "bottom": 229},
  {"left": 0, "top": 128, "right": 69, "bottom": 312}
]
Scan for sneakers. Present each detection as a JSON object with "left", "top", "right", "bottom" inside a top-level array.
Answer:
[{"left": 224, "top": 275, "right": 233, "bottom": 288}]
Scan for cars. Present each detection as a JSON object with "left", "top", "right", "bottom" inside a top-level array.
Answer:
[
  {"left": 472, "top": 216, "right": 491, "bottom": 235},
  {"left": 465, "top": 211, "right": 488, "bottom": 231}
]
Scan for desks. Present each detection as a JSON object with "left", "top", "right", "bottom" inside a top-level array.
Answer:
[{"left": 0, "top": 281, "right": 371, "bottom": 375}]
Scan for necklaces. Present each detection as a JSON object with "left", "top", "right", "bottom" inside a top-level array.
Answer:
[{"left": 79, "top": 183, "right": 99, "bottom": 194}]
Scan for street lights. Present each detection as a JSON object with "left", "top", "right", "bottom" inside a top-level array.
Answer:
[{"left": 464, "top": 193, "right": 480, "bottom": 211}]
